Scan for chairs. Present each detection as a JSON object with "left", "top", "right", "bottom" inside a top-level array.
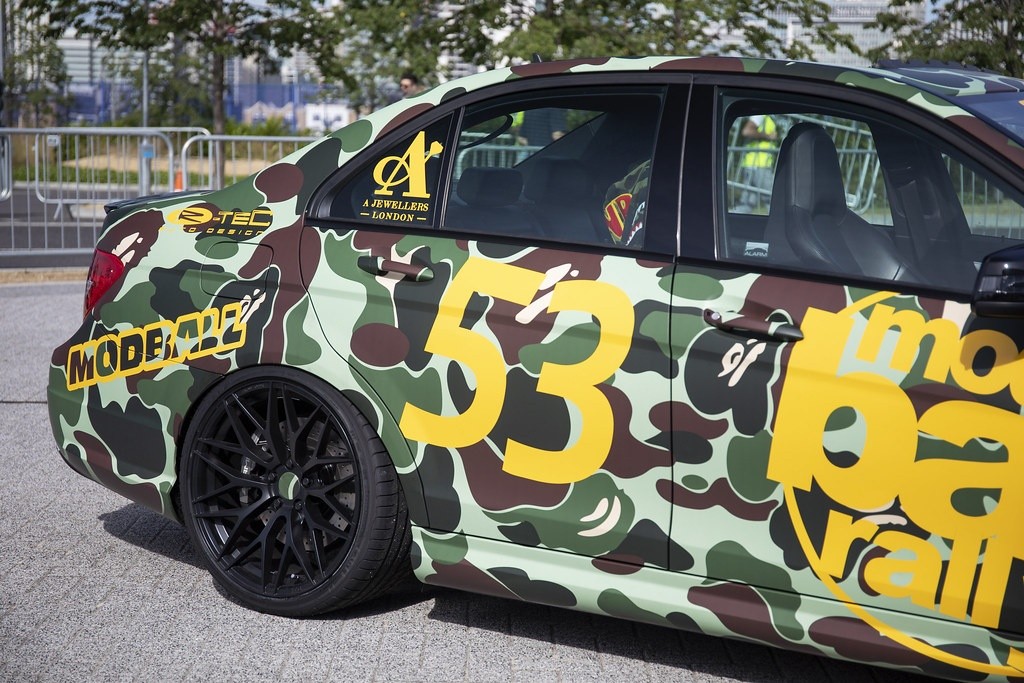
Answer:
[
  {"left": 870, "top": 120, "right": 993, "bottom": 293},
  {"left": 765, "top": 122, "right": 933, "bottom": 290},
  {"left": 445, "top": 155, "right": 610, "bottom": 244}
]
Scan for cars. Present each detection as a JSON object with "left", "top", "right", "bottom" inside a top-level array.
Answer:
[{"left": 47, "top": 50, "right": 1024, "bottom": 683}]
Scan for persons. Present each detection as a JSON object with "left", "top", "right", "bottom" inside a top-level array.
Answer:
[
  {"left": 400, "top": 72, "right": 431, "bottom": 101},
  {"left": 509, "top": 111, "right": 528, "bottom": 166},
  {"left": 737, "top": 115, "right": 778, "bottom": 215},
  {"left": 517, "top": 108, "right": 568, "bottom": 155}
]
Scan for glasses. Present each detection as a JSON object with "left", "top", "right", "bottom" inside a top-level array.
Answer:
[{"left": 399, "top": 83, "right": 408, "bottom": 89}]
